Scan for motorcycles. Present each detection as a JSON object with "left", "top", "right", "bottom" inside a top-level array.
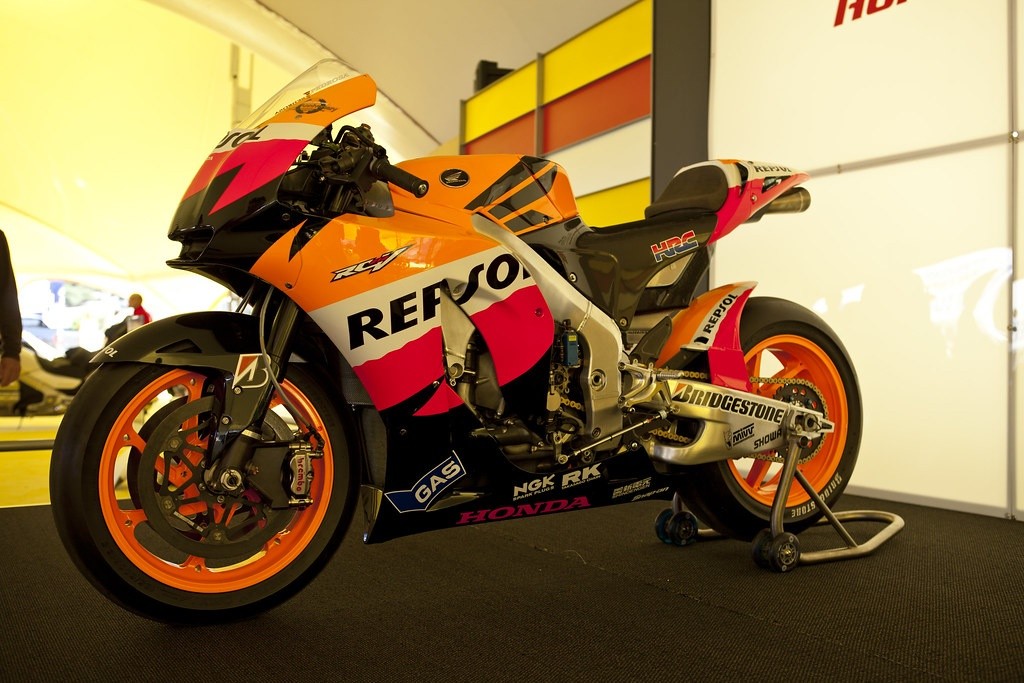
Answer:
[
  {"left": 48, "top": 56, "right": 908, "bottom": 629},
  {"left": 19, "top": 315, "right": 144, "bottom": 413}
]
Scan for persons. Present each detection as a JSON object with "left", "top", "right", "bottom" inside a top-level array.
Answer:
[
  {"left": 0, "top": 230, "right": 23, "bottom": 387},
  {"left": 129, "top": 294, "right": 152, "bottom": 325}
]
